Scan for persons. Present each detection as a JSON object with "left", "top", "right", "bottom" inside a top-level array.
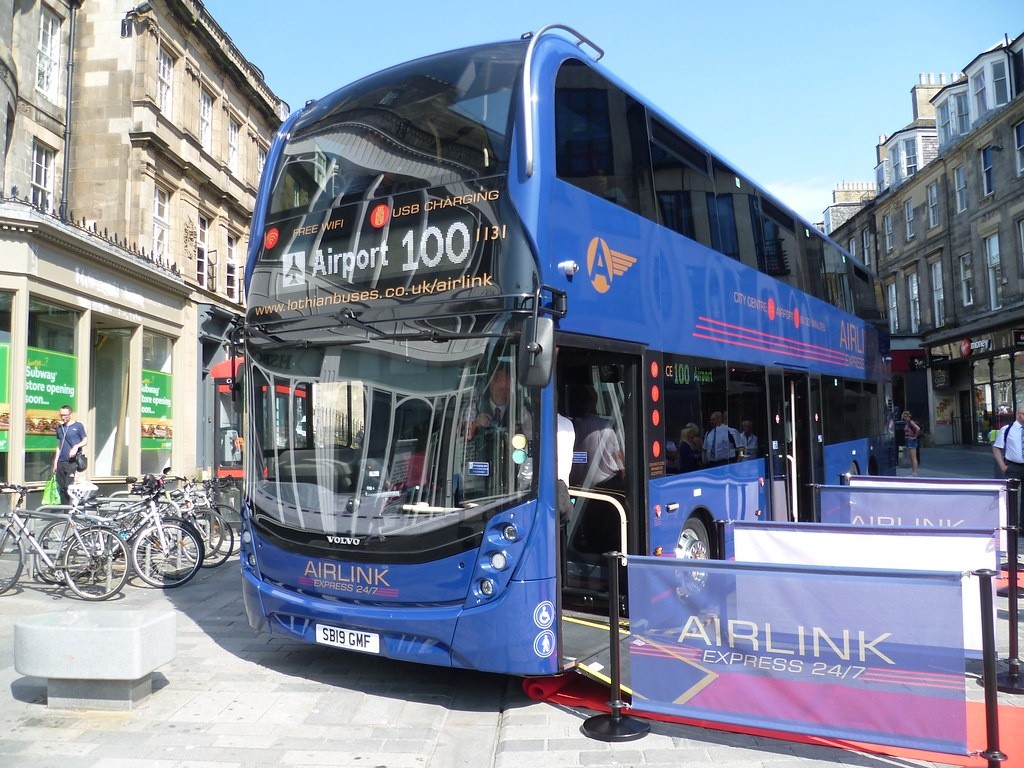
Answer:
[
  {"left": 462, "top": 369, "right": 533, "bottom": 442},
  {"left": 664, "top": 410, "right": 760, "bottom": 475},
  {"left": 902, "top": 411, "right": 920, "bottom": 476},
  {"left": 992, "top": 405, "right": 1024, "bottom": 538},
  {"left": 52, "top": 405, "right": 88, "bottom": 505},
  {"left": 556, "top": 393, "right": 628, "bottom": 554}
]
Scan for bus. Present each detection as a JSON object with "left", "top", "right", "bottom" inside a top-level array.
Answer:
[{"left": 222, "top": 24, "right": 898, "bottom": 696}]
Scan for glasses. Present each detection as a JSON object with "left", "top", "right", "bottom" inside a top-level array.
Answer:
[{"left": 60, "top": 412, "right": 72, "bottom": 417}]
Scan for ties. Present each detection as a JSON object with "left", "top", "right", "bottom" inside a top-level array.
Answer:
[
  {"left": 494, "top": 407, "right": 501, "bottom": 424},
  {"left": 710, "top": 427, "right": 716, "bottom": 460},
  {"left": 1021, "top": 425, "right": 1024, "bottom": 458}
]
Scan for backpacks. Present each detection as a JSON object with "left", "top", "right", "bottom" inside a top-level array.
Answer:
[{"left": 908, "top": 419, "right": 925, "bottom": 438}]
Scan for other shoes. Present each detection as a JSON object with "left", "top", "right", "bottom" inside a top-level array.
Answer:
[{"left": 909, "top": 472, "right": 918, "bottom": 477}]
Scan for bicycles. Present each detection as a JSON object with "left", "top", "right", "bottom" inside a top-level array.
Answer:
[{"left": 0, "top": 467, "right": 242, "bottom": 600}]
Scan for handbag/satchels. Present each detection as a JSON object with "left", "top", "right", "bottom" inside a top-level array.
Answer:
[
  {"left": 75, "top": 453, "right": 87, "bottom": 472},
  {"left": 41, "top": 473, "right": 61, "bottom": 505}
]
